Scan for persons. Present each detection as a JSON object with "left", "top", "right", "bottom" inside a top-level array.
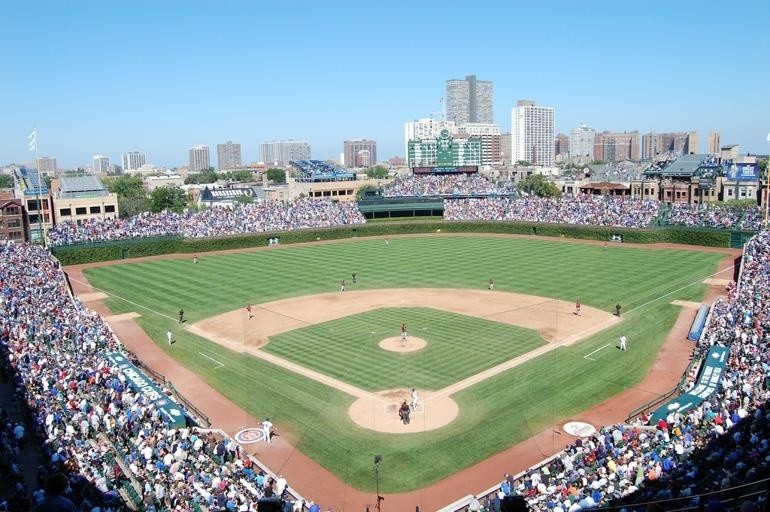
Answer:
[
  {"left": 411, "top": 388, "right": 419, "bottom": 410},
  {"left": 0, "top": 235, "right": 323, "bottom": 512},
  {"left": 575, "top": 299, "right": 582, "bottom": 316},
  {"left": 385, "top": 237, "right": 389, "bottom": 245},
  {"left": 380, "top": 173, "right": 518, "bottom": 198},
  {"left": 399, "top": 407, "right": 404, "bottom": 419},
  {"left": 468, "top": 409, "right": 679, "bottom": 512},
  {"left": 401, "top": 402, "right": 411, "bottom": 424},
  {"left": 340, "top": 280, "right": 345, "bottom": 293},
  {"left": 443, "top": 199, "right": 769, "bottom": 234},
  {"left": 488, "top": 277, "right": 494, "bottom": 289},
  {"left": 400, "top": 323, "right": 408, "bottom": 342},
  {"left": 352, "top": 272, "right": 357, "bottom": 283},
  {"left": 618, "top": 333, "right": 626, "bottom": 351},
  {"left": 616, "top": 303, "right": 622, "bottom": 317},
  {"left": 603, "top": 240, "right": 609, "bottom": 248},
  {"left": 43, "top": 197, "right": 326, "bottom": 249},
  {"left": 679, "top": 232, "right": 769, "bottom": 512},
  {"left": 322, "top": 199, "right": 368, "bottom": 228}
]
